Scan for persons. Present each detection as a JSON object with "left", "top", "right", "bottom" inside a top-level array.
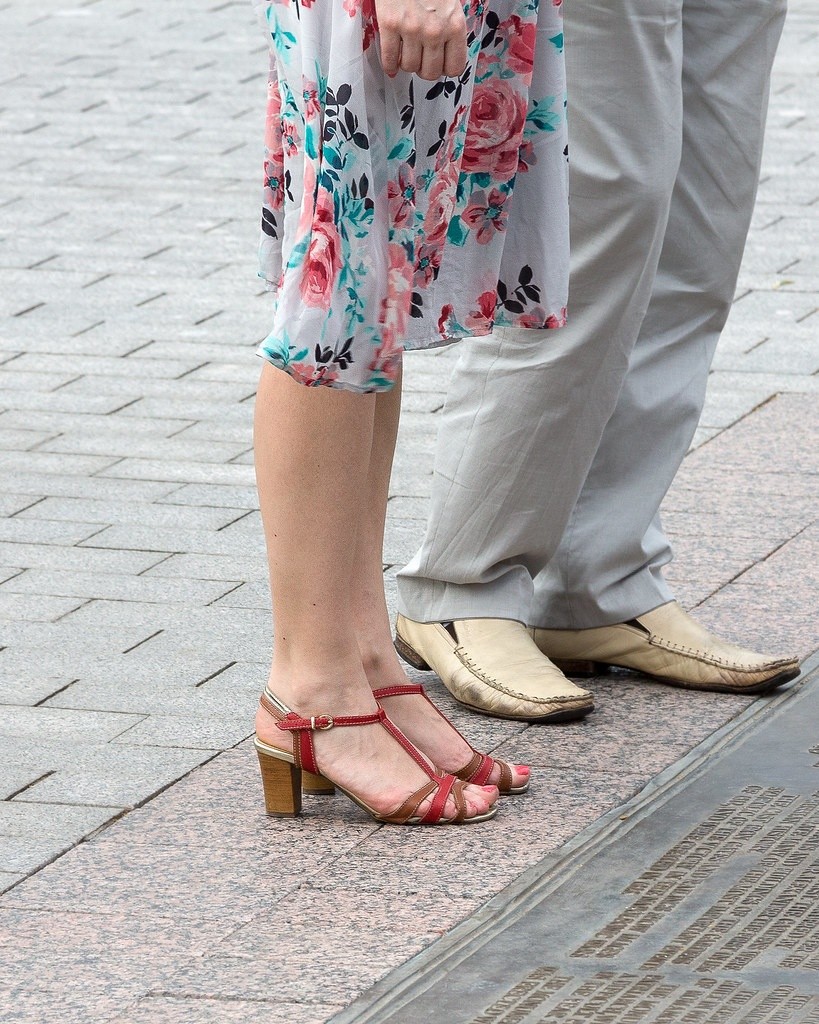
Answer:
[{"left": 249, "top": 0, "right": 803, "bottom": 827}]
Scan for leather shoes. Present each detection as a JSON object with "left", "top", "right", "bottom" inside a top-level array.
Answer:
[
  {"left": 527, "top": 600, "right": 800, "bottom": 693},
  {"left": 394, "top": 614, "right": 595, "bottom": 723}
]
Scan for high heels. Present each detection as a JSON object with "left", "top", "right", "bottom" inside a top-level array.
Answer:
[
  {"left": 253, "top": 686, "right": 498, "bottom": 824},
  {"left": 371, "top": 685, "right": 530, "bottom": 795}
]
